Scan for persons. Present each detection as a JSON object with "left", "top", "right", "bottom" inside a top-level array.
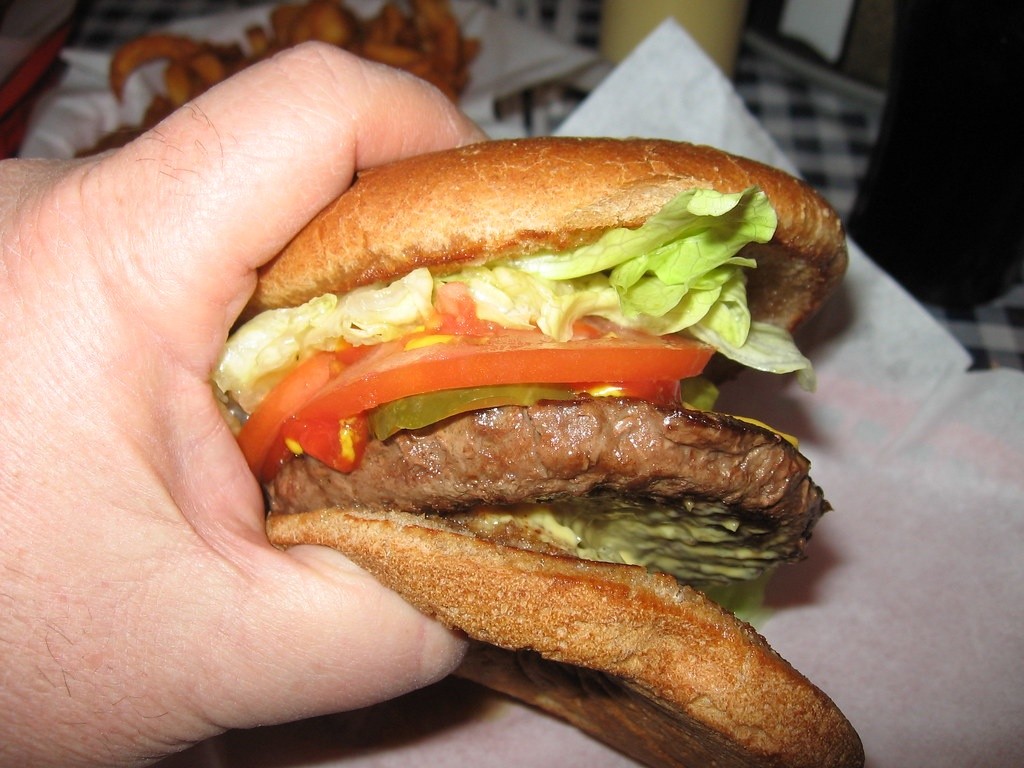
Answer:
[{"left": 0, "top": 40, "right": 494, "bottom": 766}]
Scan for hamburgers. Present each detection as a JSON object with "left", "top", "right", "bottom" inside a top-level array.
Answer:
[{"left": 216, "top": 136, "right": 869, "bottom": 768}]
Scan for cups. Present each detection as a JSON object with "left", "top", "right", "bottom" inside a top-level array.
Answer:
[{"left": 600, "top": 0, "right": 750, "bottom": 83}]
